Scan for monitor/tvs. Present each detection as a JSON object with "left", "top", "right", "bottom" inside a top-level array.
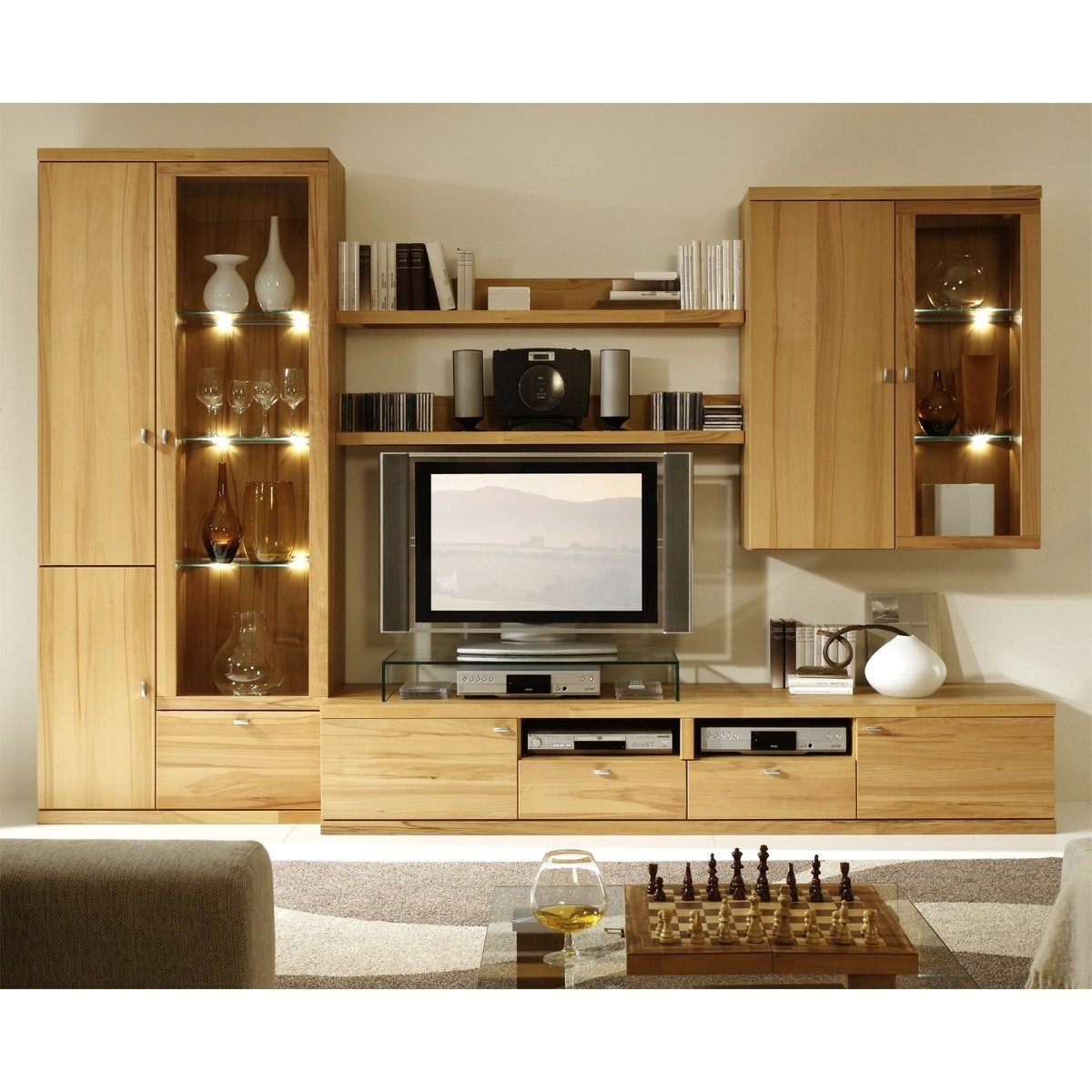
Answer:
[{"left": 378, "top": 451, "right": 694, "bottom": 656}]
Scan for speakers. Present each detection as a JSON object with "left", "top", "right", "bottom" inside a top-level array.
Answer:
[
  {"left": 601, "top": 349, "right": 632, "bottom": 430},
  {"left": 453, "top": 348, "right": 486, "bottom": 431},
  {"left": 492, "top": 349, "right": 592, "bottom": 431}
]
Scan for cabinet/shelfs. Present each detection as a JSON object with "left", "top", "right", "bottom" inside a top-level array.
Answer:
[
  {"left": 735, "top": 181, "right": 1049, "bottom": 553},
  {"left": 319, "top": 681, "right": 1059, "bottom": 841},
  {"left": 36, "top": 141, "right": 342, "bottom": 829},
  {"left": 334, "top": 306, "right": 743, "bottom": 446}
]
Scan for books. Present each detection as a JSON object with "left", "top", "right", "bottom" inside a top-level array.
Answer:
[
  {"left": 336, "top": 239, "right": 743, "bottom": 312},
  {"left": 769, "top": 617, "right": 857, "bottom": 696}
]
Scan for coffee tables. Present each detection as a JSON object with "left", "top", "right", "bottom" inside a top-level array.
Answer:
[{"left": 478, "top": 885, "right": 983, "bottom": 990}]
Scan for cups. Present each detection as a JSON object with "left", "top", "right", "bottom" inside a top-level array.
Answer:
[{"left": 243, "top": 478, "right": 297, "bottom": 564}]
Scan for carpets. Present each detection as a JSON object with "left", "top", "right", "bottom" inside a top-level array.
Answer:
[{"left": 277, "top": 854, "right": 1073, "bottom": 990}]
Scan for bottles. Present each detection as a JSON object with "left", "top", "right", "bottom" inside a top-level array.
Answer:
[
  {"left": 214, "top": 609, "right": 287, "bottom": 695},
  {"left": 200, "top": 461, "right": 243, "bottom": 562},
  {"left": 919, "top": 370, "right": 958, "bottom": 436}
]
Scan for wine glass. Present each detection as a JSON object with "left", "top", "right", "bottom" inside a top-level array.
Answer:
[
  {"left": 194, "top": 364, "right": 306, "bottom": 439},
  {"left": 529, "top": 849, "right": 608, "bottom": 965}
]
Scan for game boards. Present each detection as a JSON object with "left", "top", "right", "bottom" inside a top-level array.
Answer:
[{"left": 625, "top": 883, "right": 919, "bottom": 974}]
